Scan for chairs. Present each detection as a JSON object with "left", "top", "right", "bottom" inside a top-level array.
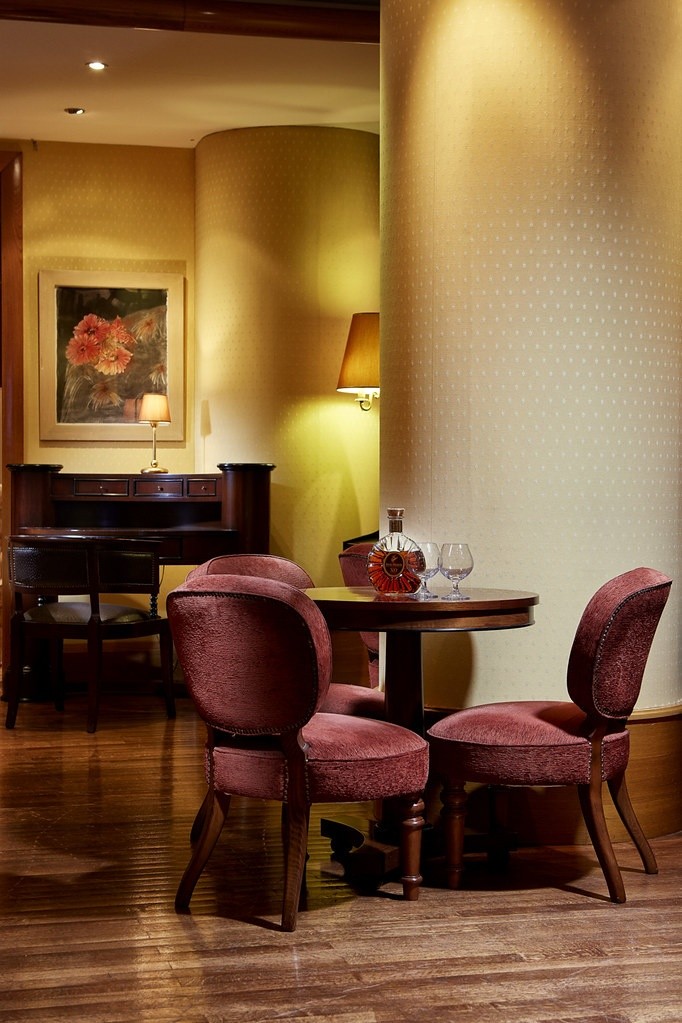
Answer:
[
  {"left": 338, "top": 541, "right": 379, "bottom": 689},
  {"left": 185, "top": 553, "right": 386, "bottom": 847},
  {"left": 425, "top": 568, "right": 672, "bottom": 905},
  {"left": 6, "top": 533, "right": 176, "bottom": 733},
  {"left": 166, "top": 574, "right": 431, "bottom": 932}
]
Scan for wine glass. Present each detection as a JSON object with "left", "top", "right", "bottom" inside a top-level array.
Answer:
[
  {"left": 439, "top": 544, "right": 474, "bottom": 600},
  {"left": 409, "top": 543, "right": 440, "bottom": 600}
]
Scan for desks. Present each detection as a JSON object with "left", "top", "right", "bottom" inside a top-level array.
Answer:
[
  {"left": 299, "top": 583, "right": 539, "bottom": 883},
  {"left": 0, "top": 462, "right": 275, "bottom": 702}
]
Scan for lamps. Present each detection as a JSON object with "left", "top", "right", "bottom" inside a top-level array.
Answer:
[
  {"left": 336, "top": 312, "right": 381, "bottom": 411},
  {"left": 137, "top": 393, "right": 172, "bottom": 475}
]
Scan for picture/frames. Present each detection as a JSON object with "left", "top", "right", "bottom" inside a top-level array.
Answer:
[{"left": 38, "top": 268, "right": 185, "bottom": 444}]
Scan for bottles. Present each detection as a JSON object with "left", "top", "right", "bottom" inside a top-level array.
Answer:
[{"left": 368, "top": 508, "right": 426, "bottom": 597}]
